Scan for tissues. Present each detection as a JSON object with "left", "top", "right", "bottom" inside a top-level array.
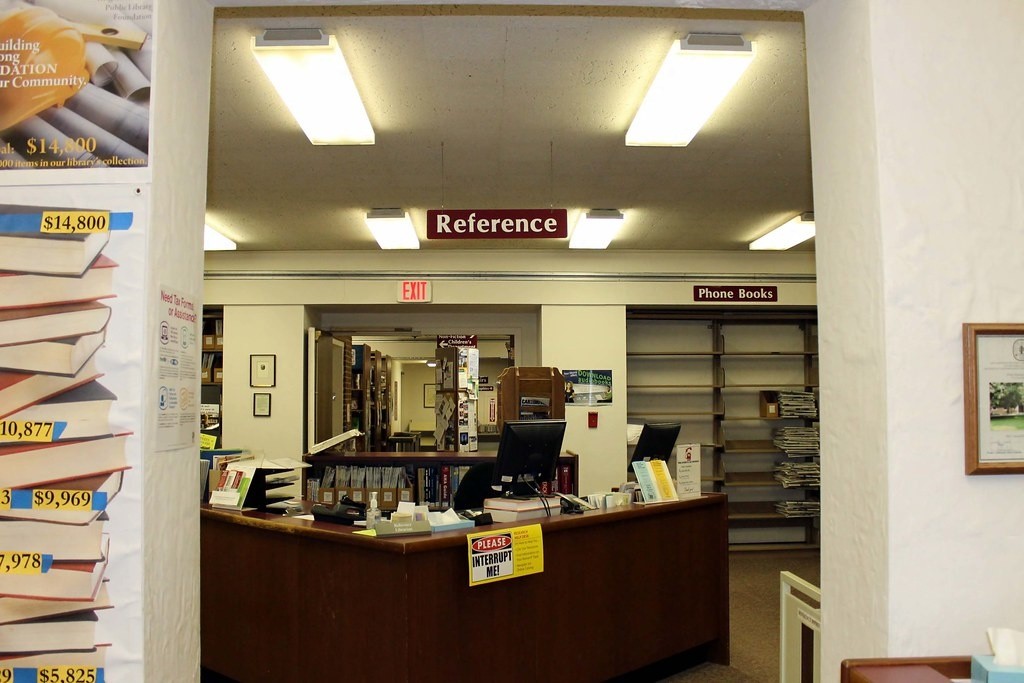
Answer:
[
  {"left": 427, "top": 507, "right": 475, "bottom": 533},
  {"left": 970, "top": 627, "right": 1024, "bottom": 683}
]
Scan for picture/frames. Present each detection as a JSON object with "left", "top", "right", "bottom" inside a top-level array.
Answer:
[
  {"left": 962, "top": 323, "right": 1024, "bottom": 476},
  {"left": 250, "top": 354, "right": 276, "bottom": 387},
  {"left": 424, "top": 384, "right": 436, "bottom": 408},
  {"left": 254, "top": 393, "right": 271, "bottom": 416}
]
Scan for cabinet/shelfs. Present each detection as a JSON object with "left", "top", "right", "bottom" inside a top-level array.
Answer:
[
  {"left": 351, "top": 344, "right": 391, "bottom": 453},
  {"left": 626, "top": 308, "right": 821, "bottom": 550},
  {"left": 497, "top": 367, "right": 565, "bottom": 434},
  {"left": 201, "top": 314, "right": 223, "bottom": 385},
  {"left": 243, "top": 468, "right": 295, "bottom": 514}
]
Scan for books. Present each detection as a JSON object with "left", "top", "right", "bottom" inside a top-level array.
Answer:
[
  {"left": 305, "top": 463, "right": 573, "bottom": 523},
  {"left": 0, "top": 204, "right": 132, "bottom": 683},
  {"left": 202, "top": 353, "right": 214, "bottom": 368},
  {"left": 199, "top": 453, "right": 257, "bottom": 507},
  {"left": 774, "top": 390, "right": 820, "bottom": 518},
  {"left": 216, "top": 320, "right": 222, "bottom": 335}
]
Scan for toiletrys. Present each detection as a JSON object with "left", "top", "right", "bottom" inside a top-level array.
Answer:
[{"left": 366, "top": 491, "right": 382, "bottom": 530}]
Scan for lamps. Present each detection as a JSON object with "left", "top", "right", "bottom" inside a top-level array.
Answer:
[
  {"left": 204, "top": 224, "right": 237, "bottom": 250},
  {"left": 748, "top": 212, "right": 816, "bottom": 250},
  {"left": 569, "top": 208, "right": 626, "bottom": 249},
  {"left": 251, "top": 28, "right": 376, "bottom": 145},
  {"left": 365, "top": 207, "right": 420, "bottom": 250},
  {"left": 625, "top": 33, "right": 757, "bottom": 148}
]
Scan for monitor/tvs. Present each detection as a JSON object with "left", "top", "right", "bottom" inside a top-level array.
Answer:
[
  {"left": 627, "top": 421, "right": 681, "bottom": 483},
  {"left": 491, "top": 418, "right": 566, "bottom": 499}
]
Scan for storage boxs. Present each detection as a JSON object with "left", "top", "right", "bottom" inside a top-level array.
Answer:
[
  {"left": 319, "top": 475, "right": 413, "bottom": 508},
  {"left": 202, "top": 368, "right": 211, "bottom": 382},
  {"left": 203, "top": 335, "right": 223, "bottom": 350},
  {"left": 214, "top": 368, "right": 222, "bottom": 383}
]
